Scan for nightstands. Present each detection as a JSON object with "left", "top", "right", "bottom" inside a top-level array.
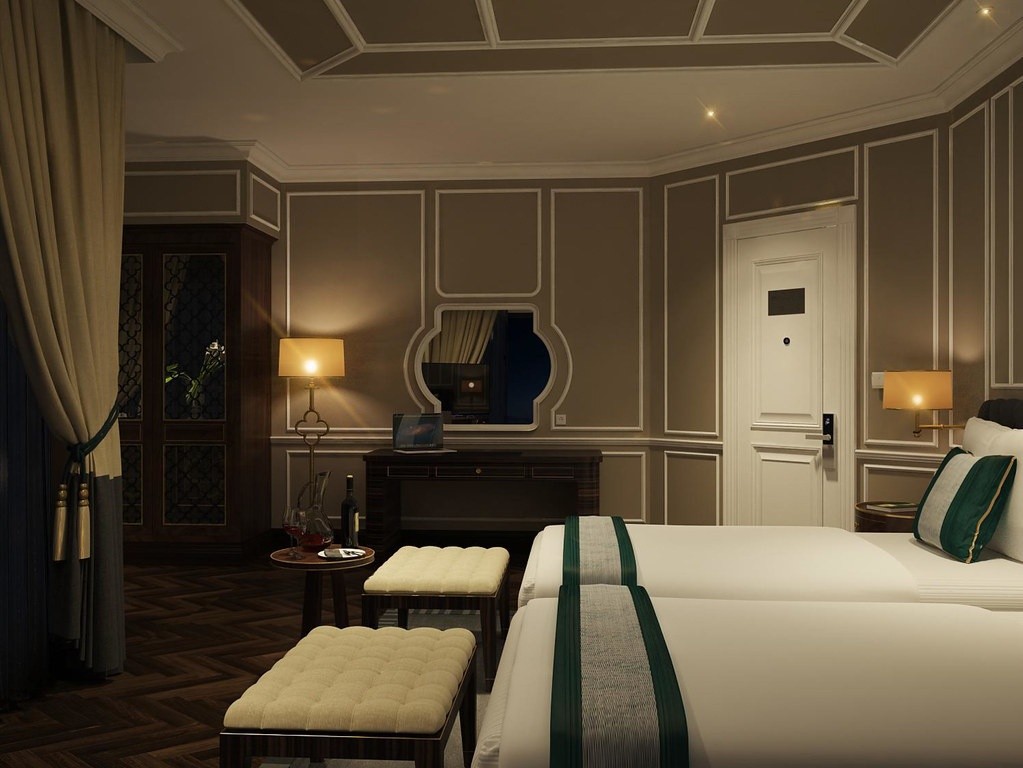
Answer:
[{"left": 854, "top": 500, "right": 918, "bottom": 533}]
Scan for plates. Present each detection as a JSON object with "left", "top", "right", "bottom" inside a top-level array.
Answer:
[{"left": 318, "top": 549, "right": 366, "bottom": 558}]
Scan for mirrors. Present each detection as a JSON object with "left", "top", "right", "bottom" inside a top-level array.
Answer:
[{"left": 413, "top": 303, "right": 558, "bottom": 431}]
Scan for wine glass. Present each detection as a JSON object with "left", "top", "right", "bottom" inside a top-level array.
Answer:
[
  {"left": 283, "top": 506, "right": 299, "bottom": 556},
  {"left": 292, "top": 510, "right": 306, "bottom": 560}
]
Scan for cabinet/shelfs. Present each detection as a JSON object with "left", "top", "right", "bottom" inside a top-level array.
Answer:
[{"left": 118, "top": 223, "right": 279, "bottom": 569}]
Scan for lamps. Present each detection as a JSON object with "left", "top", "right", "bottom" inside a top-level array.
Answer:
[
  {"left": 278, "top": 338, "right": 345, "bottom": 533},
  {"left": 883, "top": 369, "right": 966, "bottom": 439}
]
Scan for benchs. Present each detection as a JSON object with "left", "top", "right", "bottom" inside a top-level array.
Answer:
[
  {"left": 218, "top": 625, "right": 477, "bottom": 768},
  {"left": 361, "top": 545, "right": 511, "bottom": 694}
]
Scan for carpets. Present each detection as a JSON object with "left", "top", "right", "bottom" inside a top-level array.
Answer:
[{"left": 257, "top": 608, "right": 515, "bottom": 768}]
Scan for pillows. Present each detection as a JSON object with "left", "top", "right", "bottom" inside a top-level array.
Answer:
[
  {"left": 961, "top": 417, "right": 1023, "bottom": 562},
  {"left": 912, "top": 446, "right": 1017, "bottom": 564},
  {"left": 977, "top": 398, "right": 1023, "bottom": 429}
]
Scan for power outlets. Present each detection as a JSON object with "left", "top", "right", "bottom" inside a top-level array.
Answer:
[{"left": 556, "top": 415, "right": 567, "bottom": 426}]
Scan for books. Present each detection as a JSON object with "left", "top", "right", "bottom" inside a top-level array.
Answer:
[{"left": 865, "top": 502, "right": 918, "bottom": 513}]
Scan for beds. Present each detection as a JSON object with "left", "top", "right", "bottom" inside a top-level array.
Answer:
[
  {"left": 517, "top": 514, "right": 1023, "bottom": 611},
  {"left": 470, "top": 583, "right": 1023, "bottom": 768}
]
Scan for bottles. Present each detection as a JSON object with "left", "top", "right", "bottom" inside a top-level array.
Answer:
[{"left": 341, "top": 474, "right": 360, "bottom": 549}]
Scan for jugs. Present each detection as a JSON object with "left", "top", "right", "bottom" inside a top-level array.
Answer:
[{"left": 298, "top": 470, "right": 335, "bottom": 551}]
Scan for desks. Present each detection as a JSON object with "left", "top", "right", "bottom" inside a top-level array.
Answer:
[
  {"left": 269, "top": 543, "right": 375, "bottom": 639},
  {"left": 362, "top": 449, "right": 604, "bottom": 571}
]
